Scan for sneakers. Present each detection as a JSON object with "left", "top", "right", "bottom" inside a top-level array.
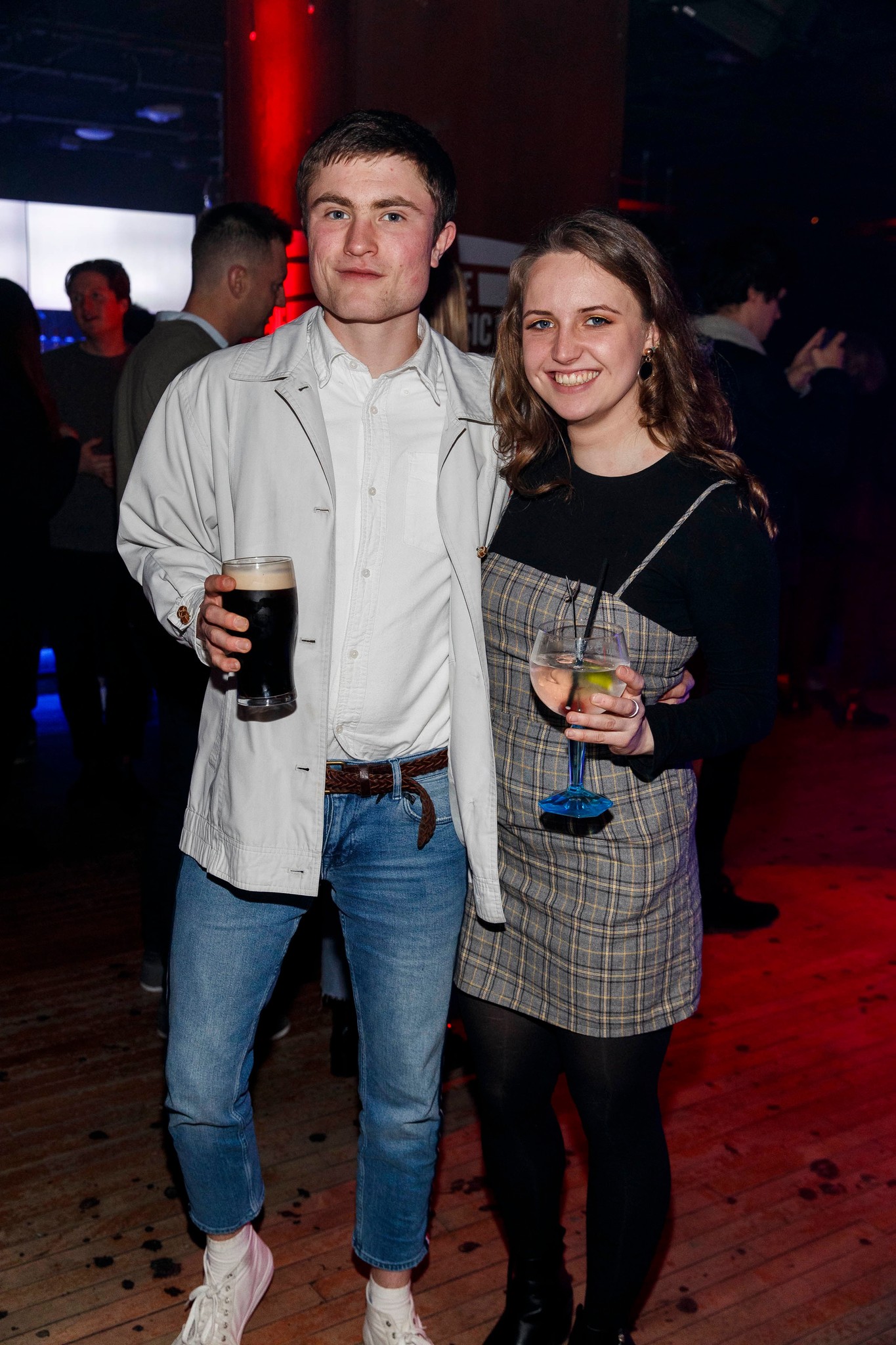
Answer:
[
  {"left": 362, "top": 1280, "right": 432, "bottom": 1345},
  {"left": 171, "top": 1225, "right": 274, "bottom": 1345}
]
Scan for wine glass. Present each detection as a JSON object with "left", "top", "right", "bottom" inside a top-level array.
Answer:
[{"left": 529, "top": 619, "right": 632, "bottom": 817}]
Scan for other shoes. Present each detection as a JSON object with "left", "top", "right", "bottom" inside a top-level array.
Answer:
[
  {"left": 140, "top": 960, "right": 164, "bottom": 994},
  {"left": 568, "top": 1303, "right": 635, "bottom": 1345},
  {"left": 701, "top": 876, "right": 779, "bottom": 935},
  {"left": 481, "top": 1283, "right": 572, "bottom": 1345}
]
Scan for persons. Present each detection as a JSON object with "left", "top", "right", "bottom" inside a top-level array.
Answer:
[
  {"left": 459, "top": 215, "right": 771, "bottom": 1345},
  {"left": 685, "top": 245, "right": 848, "bottom": 934},
  {"left": 0, "top": 260, "right": 144, "bottom": 773},
  {"left": 117, "top": 201, "right": 460, "bottom": 1073},
  {"left": 115, "top": 117, "right": 510, "bottom": 1345}
]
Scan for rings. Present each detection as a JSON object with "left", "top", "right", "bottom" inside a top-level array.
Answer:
[{"left": 626, "top": 699, "right": 638, "bottom": 718}]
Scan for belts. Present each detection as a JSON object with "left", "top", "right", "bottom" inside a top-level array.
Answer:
[{"left": 325, "top": 748, "right": 448, "bottom": 849}]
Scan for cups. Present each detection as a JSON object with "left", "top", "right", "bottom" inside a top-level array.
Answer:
[{"left": 221, "top": 556, "right": 298, "bottom": 707}]
